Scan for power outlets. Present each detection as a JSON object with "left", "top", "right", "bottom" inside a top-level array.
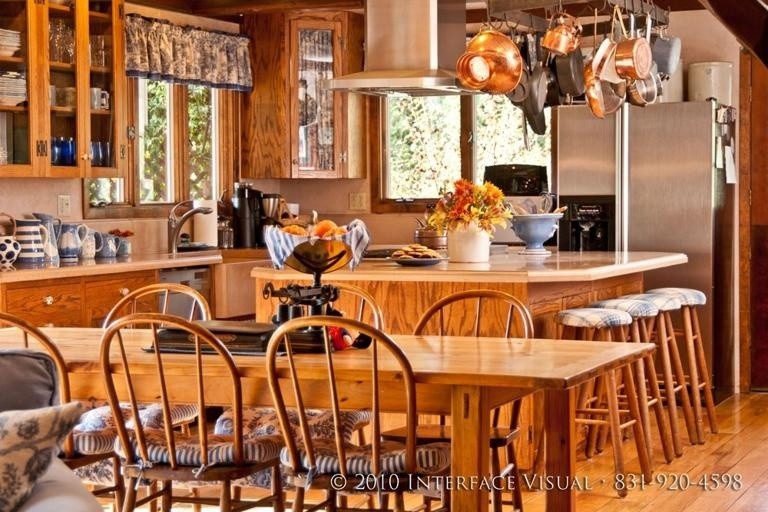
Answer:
[
  {"left": 57, "top": 195, "right": 72, "bottom": 218},
  {"left": 348, "top": 191, "right": 367, "bottom": 211}
]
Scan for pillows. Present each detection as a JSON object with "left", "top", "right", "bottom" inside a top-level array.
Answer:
[{"left": 0, "top": 400, "right": 87, "bottom": 512}]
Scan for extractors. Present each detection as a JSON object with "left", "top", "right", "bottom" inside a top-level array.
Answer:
[{"left": 327, "top": 0, "right": 478, "bottom": 97}]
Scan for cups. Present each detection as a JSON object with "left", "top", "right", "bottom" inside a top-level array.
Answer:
[
  {"left": 99, "top": 236, "right": 121, "bottom": 258},
  {"left": 58, "top": 223, "right": 88, "bottom": 262},
  {"left": 15, "top": 217, "right": 48, "bottom": 265},
  {"left": 79, "top": 228, "right": 104, "bottom": 259},
  {"left": 49, "top": 136, "right": 112, "bottom": 166},
  {"left": 48, "top": 83, "right": 78, "bottom": 107},
  {"left": 90, "top": 88, "right": 108, "bottom": 110}
]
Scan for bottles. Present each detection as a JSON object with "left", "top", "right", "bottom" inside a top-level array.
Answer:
[
  {"left": 218, "top": 220, "right": 234, "bottom": 249},
  {"left": 51, "top": 136, "right": 76, "bottom": 164},
  {"left": 101, "top": 88, "right": 106, "bottom": 106}
]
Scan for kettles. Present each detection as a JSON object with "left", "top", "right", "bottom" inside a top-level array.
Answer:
[{"left": 231, "top": 181, "right": 263, "bottom": 249}]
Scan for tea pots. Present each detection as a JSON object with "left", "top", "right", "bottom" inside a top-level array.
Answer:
[
  {"left": 32, "top": 210, "right": 61, "bottom": 262},
  {"left": 0, "top": 212, "right": 21, "bottom": 273},
  {"left": 540, "top": 11, "right": 583, "bottom": 57}
]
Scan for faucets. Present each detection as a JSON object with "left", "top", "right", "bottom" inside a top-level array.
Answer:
[{"left": 167, "top": 200, "right": 213, "bottom": 254}]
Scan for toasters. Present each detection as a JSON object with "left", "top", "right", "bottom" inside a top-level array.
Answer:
[{"left": 483, "top": 164, "right": 548, "bottom": 196}]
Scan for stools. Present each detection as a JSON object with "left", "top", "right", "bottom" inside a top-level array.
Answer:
[{"left": 552, "top": 287, "right": 719, "bottom": 498}]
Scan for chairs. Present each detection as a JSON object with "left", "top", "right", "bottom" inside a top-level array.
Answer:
[
  {"left": 380, "top": 289, "right": 535, "bottom": 512},
  {"left": 214, "top": 282, "right": 451, "bottom": 512},
  {"left": 101, "top": 282, "right": 286, "bottom": 512},
  {"left": 0, "top": 311, "right": 136, "bottom": 512}
]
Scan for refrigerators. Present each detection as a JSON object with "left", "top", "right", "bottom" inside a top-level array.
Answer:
[{"left": 558, "top": 101, "right": 716, "bottom": 407}]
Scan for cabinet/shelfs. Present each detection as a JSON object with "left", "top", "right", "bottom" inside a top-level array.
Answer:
[
  {"left": 0, "top": 269, "right": 160, "bottom": 329},
  {"left": 0, "top": 0, "right": 129, "bottom": 179},
  {"left": 240, "top": 8, "right": 368, "bottom": 180}
]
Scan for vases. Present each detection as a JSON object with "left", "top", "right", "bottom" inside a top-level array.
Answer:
[{"left": 447, "top": 219, "right": 490, "bottom": 263}]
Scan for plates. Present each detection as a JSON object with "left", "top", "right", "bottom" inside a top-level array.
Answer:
[{"left": 385, "top": 258, "right": 442, "bottom": 266}]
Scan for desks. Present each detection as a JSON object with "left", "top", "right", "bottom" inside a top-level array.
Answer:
[
  {"left": 0, "top": 324, "right": 656, "bottom": 512},
  {"left": 249, "top": 251, "right": 690, "bottom": 493}
]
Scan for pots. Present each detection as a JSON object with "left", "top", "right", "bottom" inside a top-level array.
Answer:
[
  {"left": 414, "top": 216, "right": 448, "bottom": 248},
  {"left": 505, "top": 30, "right": 584, "bottom": 135},
  {"left": 583, "top": 9, "right": 682, "bottom": 120}
]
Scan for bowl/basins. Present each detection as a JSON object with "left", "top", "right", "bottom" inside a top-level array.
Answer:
[
  {"left": 264, "top": 220, "right": 369, "bottom": 275},
  {"left": 456, "top": 30, "right": 523, "bottom": 96}
]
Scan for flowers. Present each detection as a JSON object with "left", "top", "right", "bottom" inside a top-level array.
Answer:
[{"left": 427, "top": 178, "right": 513, "bottom": 239}]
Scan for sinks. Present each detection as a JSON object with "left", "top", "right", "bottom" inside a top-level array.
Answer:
[{"left": 173, "top": 249, "right": 271, "bottom": 264}]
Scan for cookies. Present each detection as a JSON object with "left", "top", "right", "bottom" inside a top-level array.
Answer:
[{"left": 392, "top": 243, "right": 439, "bottom": 259}]
